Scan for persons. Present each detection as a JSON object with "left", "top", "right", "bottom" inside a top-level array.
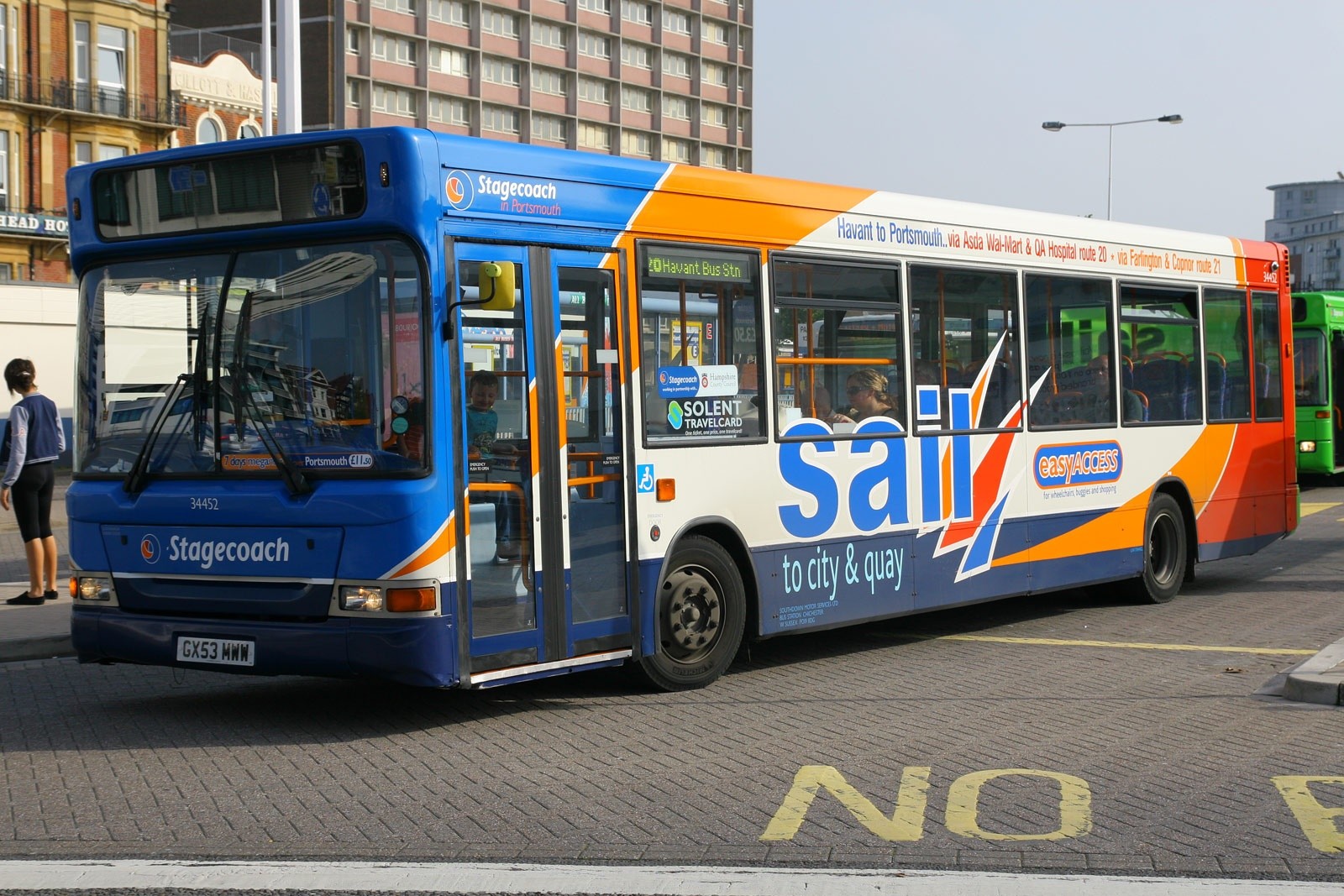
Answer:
[
  {"left": 644, "top": 354, "right": 1141, "bottom": 430},
  {"left": 384, "top": 369, "right": 525, "bottom": 565},
  {"left": 0, "top": 357, "right": 66, "bottom": 605}
]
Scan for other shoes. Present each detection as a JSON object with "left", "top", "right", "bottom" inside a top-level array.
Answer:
[
  {"left": 44, "top": 589, "right": 58, "bottom": 600},
  {"left": 5, "top": 590, "right": 47, "bottom": 605}
]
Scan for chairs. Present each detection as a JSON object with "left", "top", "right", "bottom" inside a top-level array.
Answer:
[{"left": 929, "top": 351, "right": 1273, "bottom": 422}]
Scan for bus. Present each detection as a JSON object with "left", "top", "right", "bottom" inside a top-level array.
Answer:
[
  {"left": 811, "top": 310, "right": 1016, "bottom": 423},
  {"left": 1055, "top": 291, "right": 1344, "bottom": 479},
  {"left": 64, "top": 126, "right": 1301, "bottom": 692}
]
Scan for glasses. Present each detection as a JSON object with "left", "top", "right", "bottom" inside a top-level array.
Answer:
[{"left": 846, "top": 386, "right": 866, "bottom": 394}]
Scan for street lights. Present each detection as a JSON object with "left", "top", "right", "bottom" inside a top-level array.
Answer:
[{"left": 1042, "top": 114, "right": 1183, "bottom": 221}]
